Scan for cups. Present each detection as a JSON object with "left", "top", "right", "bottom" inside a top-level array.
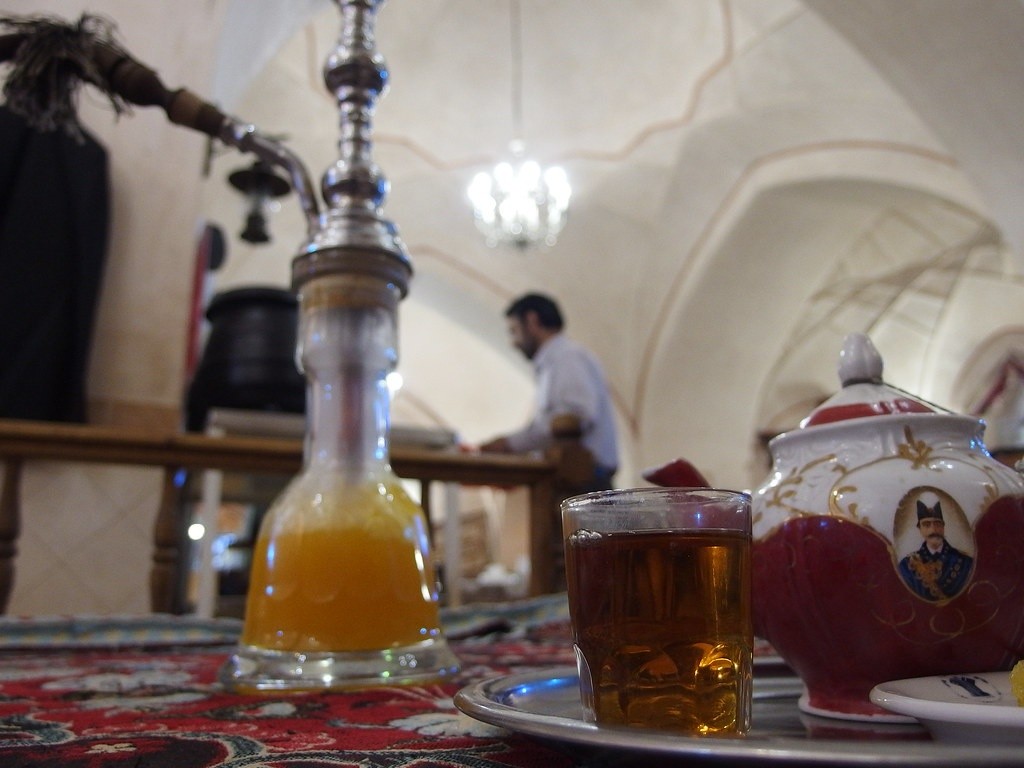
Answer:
[{"left": 560, "top": 486, "right": 755, "bottom": 744}]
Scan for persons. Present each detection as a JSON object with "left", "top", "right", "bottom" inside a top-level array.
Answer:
[{"left": 480, "top": 292, "right": 618, "bottom": 498}]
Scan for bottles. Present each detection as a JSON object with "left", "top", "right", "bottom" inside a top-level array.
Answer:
[{"left": 750, "top": 329, "right": 1024, "bottom": 724}]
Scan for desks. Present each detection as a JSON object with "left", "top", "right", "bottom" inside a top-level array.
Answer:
[{"left": 0, "top": 415, "right": 560, "bottom": 635}]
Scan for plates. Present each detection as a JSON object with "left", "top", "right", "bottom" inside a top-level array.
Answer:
[{"left": 868, "top": 670, "right": 1024, "bottom": 748}]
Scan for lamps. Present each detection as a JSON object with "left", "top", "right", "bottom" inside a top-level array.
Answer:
[{"left": 465, "top": 0, "right": 572, "bottom": 248}]
